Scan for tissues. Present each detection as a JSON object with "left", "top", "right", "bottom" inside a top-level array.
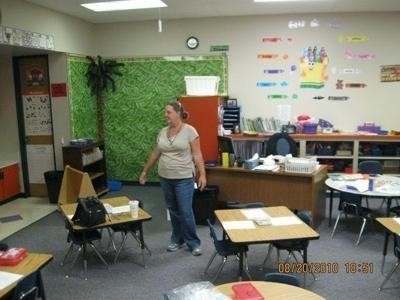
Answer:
[{"left": 243, "top": 152, "right": 261, "bottom": 169}]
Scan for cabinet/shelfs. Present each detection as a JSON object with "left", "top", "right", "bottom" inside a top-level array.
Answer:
[
  {"left": 62, "top": 139, "right": 108, "bottom": 198},
  {"left": 222, "top": 131, "right": 400, "bottom": 174},
  {"left": 0, "top": 159, "right": 22, "bottom": 206},
  {"left": 178, "top": 94, "right": 229, "bottom": 162}
]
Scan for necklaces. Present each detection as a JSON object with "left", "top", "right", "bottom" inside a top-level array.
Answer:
[{"left": 168, "top": 122, "right": 183, "bottom": 145}]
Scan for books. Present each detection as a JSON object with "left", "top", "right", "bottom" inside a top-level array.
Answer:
[
  {"left": 233, "top": 141, "right": 268, "bottom": 162},
  {"left": 241, "top": 116, "right": 282, "bottom": 133}
]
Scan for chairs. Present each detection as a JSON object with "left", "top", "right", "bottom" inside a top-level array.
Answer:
[
  {"left": 228, "top": 201, "right": 280, "bottom": 272},
  {"left": 256, "top": 210, "right": 317, "bottom": 282},
  {"left": 61, "top": 216, "right": 111, "bottom": 279},
  {"left": 204, "top": 218, "right": 252, "bottom": 284},
  {"left": 106, "top": 198, "right": 151, "bottom": 264},
  {"left": 330, "top": 192, "right": 375, "bottom": 246},
  {"left": 265, "top": 133, "right": 297, "bottom": 157},
  {"left": 359, "top": 161, "right": 383, "bottom": 174},
  {"left": 264, "top": 273, "right": 304, "bottom": 288}
]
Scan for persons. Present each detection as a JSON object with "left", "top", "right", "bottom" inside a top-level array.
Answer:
[{"left": 139, "top": 101, "right": 207, "bottom": 256}]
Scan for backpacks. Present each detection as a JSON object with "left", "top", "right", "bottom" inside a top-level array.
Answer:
[{"left": 71, "top": 196, "right": 109, "bottom": 228}]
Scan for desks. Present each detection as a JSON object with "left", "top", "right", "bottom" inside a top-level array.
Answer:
[
  {"left": 61, "top": 195, "right": 152, "bottom": 280},
  {"left": 213, "top": 205, "right": 317, "bottom": 283},
  {"left": 211, "top": 280, "right": 327, "bottom": 300},
  {"left": 196, "top": 163, "right": 329, "bottom": 231},
  {"left": 374, "top": 217, "right": 400, "bottom": 294},
  {"left": 0, "top": 253, "right": 54, "bottom": 300},
  {"left": 324, "top": 174, "right": 400, "bottom": 229}
]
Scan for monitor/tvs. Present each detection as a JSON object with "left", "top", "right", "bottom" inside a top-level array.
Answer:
[{"left": 217, "top": 136, "right": 236, "bottom": 165}]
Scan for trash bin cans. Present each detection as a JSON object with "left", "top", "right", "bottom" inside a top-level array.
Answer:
[
  {"left": 192, "top": 185, "right": 219, "bottom": 225},
  {"left": 44, "top": 170, "right": 64, "bottom": 203}
]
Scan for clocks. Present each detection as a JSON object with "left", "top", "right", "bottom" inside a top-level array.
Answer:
[{"left": 186, "top": 36, "right": 199, "bottom": 49}]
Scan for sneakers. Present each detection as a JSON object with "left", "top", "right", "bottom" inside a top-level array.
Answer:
[
  {"left": 190, "top": 246, "right": 204, "bottom": 256},
  {"left": 166, "top": 242, "right": 186, "bottom": 252}
]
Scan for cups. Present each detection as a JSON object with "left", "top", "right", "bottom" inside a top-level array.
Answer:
[
  {"left": 128, "top": 200, "right": 139, "bottom": 218},
  {"left": 368, "top": 177, "right": 375, "bottom": 191},
  {"left": 222, "top": 152, "right": 235, "bottom": 168}
]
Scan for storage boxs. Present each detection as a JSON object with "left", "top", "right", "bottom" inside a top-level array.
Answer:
[
  {"left": 307, "top": 146, "right": 352, "bottom": 156},
  {"left": 184, "top": 75, "right": 219, "bottom": 96}
]
